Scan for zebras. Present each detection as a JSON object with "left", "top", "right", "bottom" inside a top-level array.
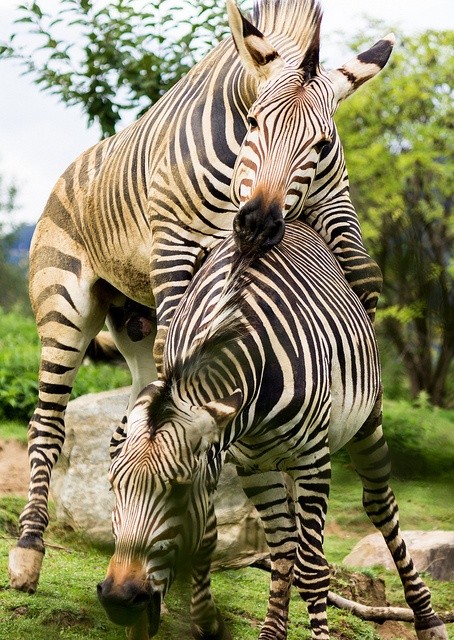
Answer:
[
  {"left": 94, "top": 218, "right": 449, "bottom": 640},
  {"left": 6, "top": 0, "right": 387, "bottom": 593}
]
